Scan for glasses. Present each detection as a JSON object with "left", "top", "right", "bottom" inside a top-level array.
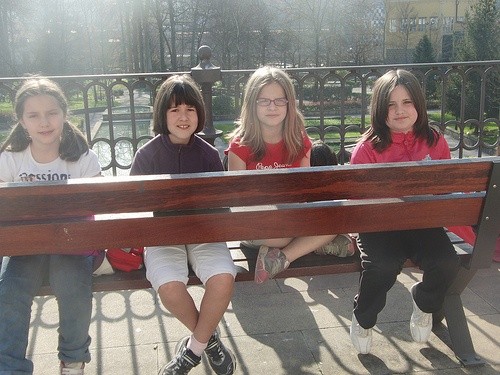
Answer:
[{"left": 256, "top": 98, "right": 288, "bottom": 106}]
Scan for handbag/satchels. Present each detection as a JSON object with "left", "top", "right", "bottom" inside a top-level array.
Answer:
[{"left": 106, "top": 247, "right": 143, "bottom": 272}]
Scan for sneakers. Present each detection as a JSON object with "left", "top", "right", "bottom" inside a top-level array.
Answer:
[
  {"left": 202, "top": 325, "right": 237, "bottom": 375},
  {"left": 158, "top": 336, "right": 201, "bottom": 375},
  {"left": 314, "top": 234, "right": 355, "bottom": 258},
  {"left": 350, "top": 312, "right": 372, "bottom": 354},
  {"left": 255, "top": 246, "right": 290, "bottom": 286},
  {"left": 410, "top": 299, "right": 433, "bottom": 344}
]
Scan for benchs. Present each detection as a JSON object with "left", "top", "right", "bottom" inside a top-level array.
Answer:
[{"left": 0, "top": 157, "right": 499, "bottom": 367}]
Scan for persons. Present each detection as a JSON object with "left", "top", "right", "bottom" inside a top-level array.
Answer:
[
  {"left": 350, "top": 70, "right": 457, "bottom": 355},
  {"left": 129, "top": 74, "right": 238, "bottom": 375},
  {"left": 223, "top": 65, "right": 354, "bottom": 284},
  {"left": 0, "top": 78, "right": 102, "bottom": 375}
]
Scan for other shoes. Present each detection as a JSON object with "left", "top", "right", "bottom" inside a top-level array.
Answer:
[{"left": 60, "top": 361, "right": 85, "bottom": 375}]
